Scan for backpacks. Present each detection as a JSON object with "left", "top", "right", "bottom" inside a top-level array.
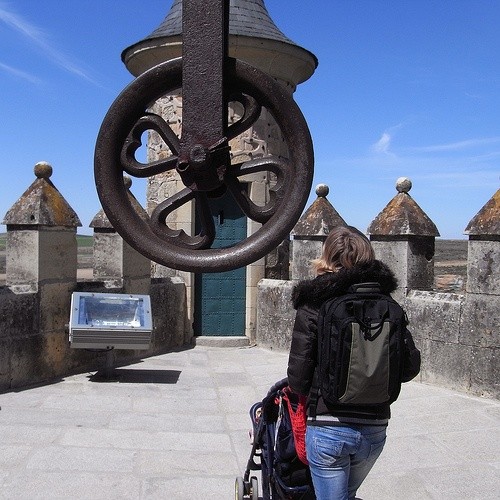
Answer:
[{"left": 313, "top": 282, "right": 405, "bottom": 411}]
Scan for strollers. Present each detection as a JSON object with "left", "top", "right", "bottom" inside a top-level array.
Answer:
[{"left": 234, "top": 375, "right": 317, "bottom": 500}]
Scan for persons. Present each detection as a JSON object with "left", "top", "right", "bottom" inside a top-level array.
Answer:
[{"left": 288, "top": 224, "right": 421, "bottom": 500}]
